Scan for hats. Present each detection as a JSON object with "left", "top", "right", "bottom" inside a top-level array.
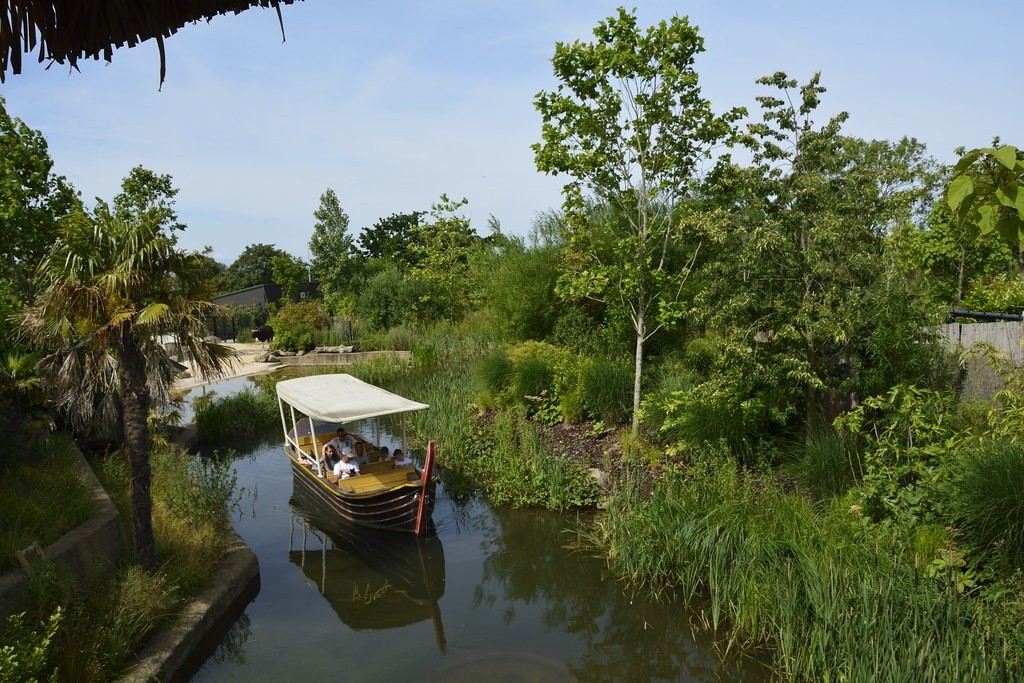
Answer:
[{"left": 341, "top": 447, "right": 355, "bottom": 458}]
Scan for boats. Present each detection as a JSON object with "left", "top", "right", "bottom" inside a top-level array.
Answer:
[{"left": 274, "top": 373, "right": 440, "bottom": 533}]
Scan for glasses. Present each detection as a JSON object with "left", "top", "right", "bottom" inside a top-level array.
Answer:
[{"left": 338, "top": 433, "right": 347, "bottom": 437}]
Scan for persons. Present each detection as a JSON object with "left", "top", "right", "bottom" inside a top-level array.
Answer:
[
  {"left": 334, "top": 447, "right": 362, "bottom": 484},
  {"left": 320, "top": 446, "right": 341, "bottom": 471},
  {"left": 379, "top": 447, "right": 392, "bottom": 463},
  {"left": 353, "top": 442, "right": 369, "bottom": 465},
  {"left": 392, "top": 449, "right": 413, "bottom": 469},
  {"left": 322, "top": 427, "right": 357, "bottom": 457}
]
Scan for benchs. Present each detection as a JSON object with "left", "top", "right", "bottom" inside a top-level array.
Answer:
[
  {"left": 310, "top": 443, "right": 373, "bottom": 467},
  {"left": 327, "top": 460, "right": 395, "bottom": 487},
  {"left": 298, "top": 434, "right": 364, "bottom": 462},
  {"left": 293, "top": 432, "right": 351, "bottom": 453},
  {"left": 321, "top": 450, "right": 384, "bottom": 477}
]
